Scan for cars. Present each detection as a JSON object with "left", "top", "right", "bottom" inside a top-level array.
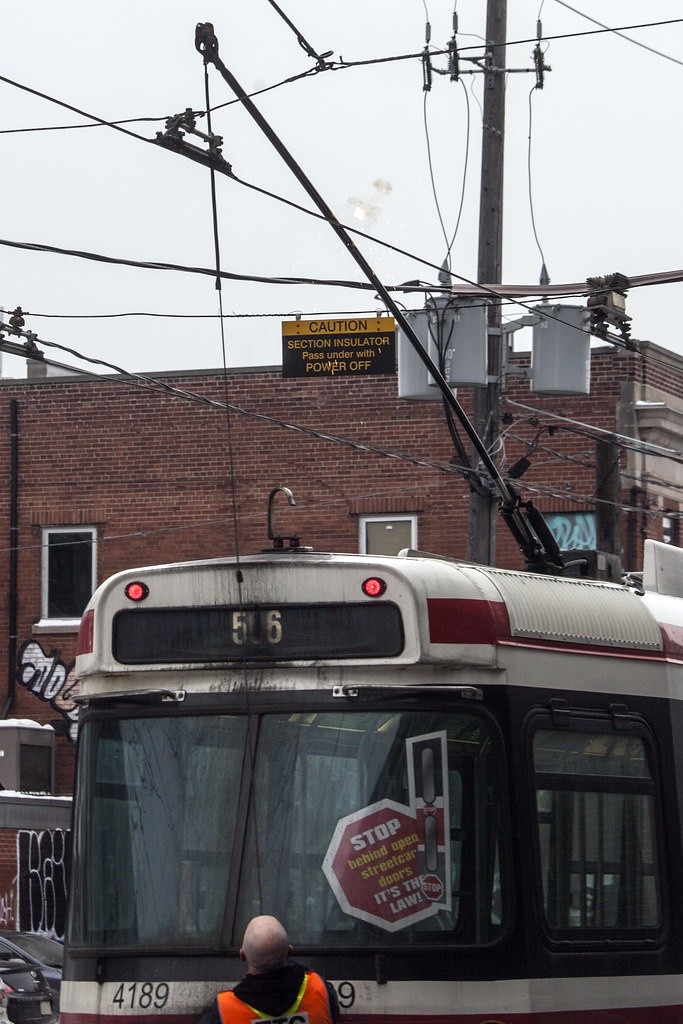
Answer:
[{"left": 0, "top": 929, "right": 64, "bottom": 1024}]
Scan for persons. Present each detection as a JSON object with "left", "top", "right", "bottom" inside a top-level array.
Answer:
[{"left": 197, "top": 915, "right": 340, "bottom": 1023}]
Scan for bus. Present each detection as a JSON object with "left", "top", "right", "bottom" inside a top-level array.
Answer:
[{"left": 59, "top": 545, "right": 683, "bottom": 1024}]
